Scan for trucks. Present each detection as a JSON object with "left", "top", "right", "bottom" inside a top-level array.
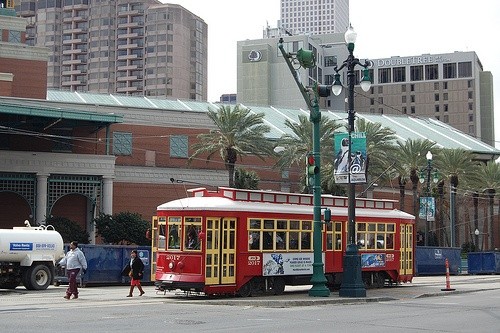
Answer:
[{"left": 0, "top": 220, "right": 65, "bottom": 289}]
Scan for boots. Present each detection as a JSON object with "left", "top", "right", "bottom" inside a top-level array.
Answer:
[
  {"left": 137, "top": 285, "right": 145, "bottom": 296},
  {"left": 126, "top": 285, "right": 134, "bottom": 297}
]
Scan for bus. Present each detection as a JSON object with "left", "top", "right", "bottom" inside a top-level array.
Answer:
[{"left": 145, "top": 185, "right": 416, "bottom": 298}]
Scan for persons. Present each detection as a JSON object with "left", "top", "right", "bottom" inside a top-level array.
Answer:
[
  {"left": 56, "top": 241, "right": 88, "bottom": 300},
  {"left": 127, "top": 250, "right": 145, "bottom": 297}
]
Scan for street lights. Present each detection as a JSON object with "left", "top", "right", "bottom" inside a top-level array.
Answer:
[
  {"left": 418, "top": 151, "right": 438, "bottom": 246},
  {"left": 474, "top": 228, "right": 480, "bottom": 252},
  {"left": 331, "top": 22, "right": 374, "bottom": 300}
]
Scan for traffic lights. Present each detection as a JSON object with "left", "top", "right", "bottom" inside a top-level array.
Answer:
[
  {"left": 298, "top": 48, "right": 315, "bottom": 69},
  {"left": 305, "top": 155, "right": 319, "bottom": 186},
  {"left": 324, "top": 208, "right": 331, "bottom": 223},
  {"left": 318, "top": 84, "right": 332, "bottom": 97}
]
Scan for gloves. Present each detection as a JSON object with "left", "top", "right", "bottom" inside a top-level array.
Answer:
[
  {"left": 56, "top": 263, "right": 60, "bottom": 267},
  {"left": 83, "top": 269, "right": 87, "bottom": 275}
]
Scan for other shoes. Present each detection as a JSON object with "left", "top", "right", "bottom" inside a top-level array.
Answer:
[
  {"left": 72, "top": 296, "right": 78, "bottom": 299},
  {"left": 64, "top": 295, "right": 70, "bottom": 299}
]
